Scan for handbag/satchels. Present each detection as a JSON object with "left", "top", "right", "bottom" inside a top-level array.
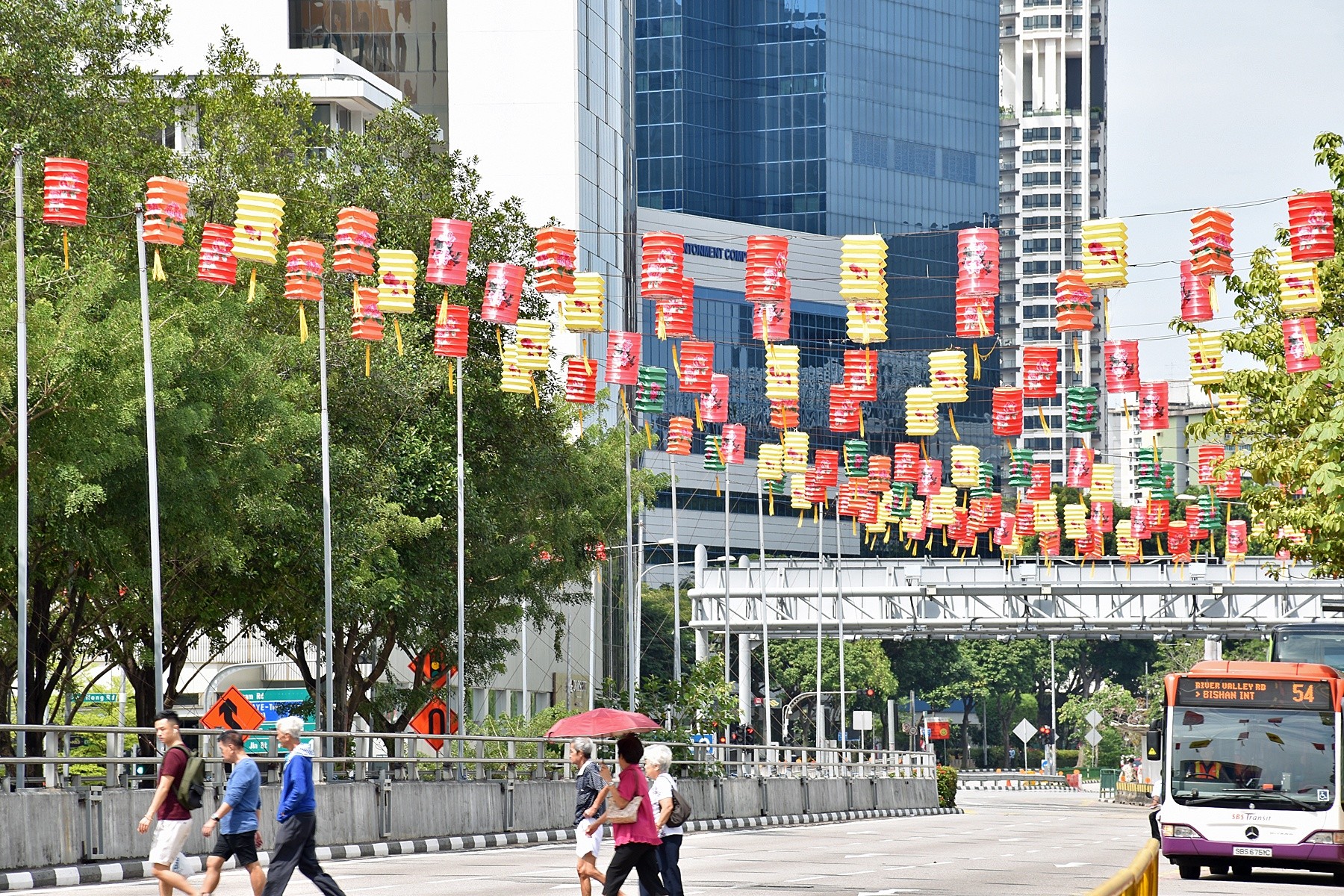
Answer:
[
  {"left": 606, "top": 796, "right": 643, "bottom": 824},
  {"left": 658, "top": 788, "right": 691, "bottom": 828},
  {"left": 168, "top": 852, "right": 195, "bottom": 879}
]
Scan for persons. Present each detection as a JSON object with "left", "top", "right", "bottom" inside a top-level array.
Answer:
[
  {"left": 569, "top": 737, "right": 627, "bottom": 896},
  {"left": 953, "top": 746, "right": 1015, "bottom": 769},
  {"left": 261, "top": 716, "right": 346, "bottom": 896},
  {"left": 138, "top": 710, "right": 201, "bottom": 896},
  {"left": 1186, "top": 742, "right": 1232, "bottom": 783},
  {"left": 639, "top": 746, "right": 684, "bottom": 896},
  {"left": 201, "top": 730, "right": 266, "bottom": 896},
  {"left": 1119, "top": 757, "right": 1143, "bottom": 783},
  {"left": 598, "top": 733, "right": 667, "bottom": 896},
  {"left": 1148, "top": 769, "right": 1162, "bottom": 851}
]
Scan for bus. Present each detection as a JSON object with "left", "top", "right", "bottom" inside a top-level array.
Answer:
[
  {"left": 1145, "top": 659, "right": 1344, "bottom": 886},
  {"left": 1269, "top": 622, "right": 1344, "bottom": 676}
]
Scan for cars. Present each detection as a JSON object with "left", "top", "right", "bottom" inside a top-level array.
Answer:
[{"left": 1121, "top": 758, "right": 1143, "bottom": 774}]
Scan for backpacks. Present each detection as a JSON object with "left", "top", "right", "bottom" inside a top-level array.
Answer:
[{"left": 160, "top": 746, "right": 206, "bottom": 811}]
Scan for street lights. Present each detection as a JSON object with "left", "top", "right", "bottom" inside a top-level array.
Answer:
[
  {"left": 781, "top": 704, "right": 793, "bottom": 741},
  {"left": 633, "top": 555, "right": 736, "bottom": 708},
  {"left": 521, "top": 538, "right": 687, "bottom": 731}
]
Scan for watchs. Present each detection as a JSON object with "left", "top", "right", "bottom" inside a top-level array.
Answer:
[
  {"left": 210, "top": 814, "right": 220, "bottom": 821},
  {"left": 608, "top": 782, "right": 614, "bottom": 786}
]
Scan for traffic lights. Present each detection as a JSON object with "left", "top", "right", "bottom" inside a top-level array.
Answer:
[
  {"left": 1044, "top": 729, "right": 1055, "bottom": 745},
  {"left": 735, "top": 724, "right": 745, "bottom": 745},
  {"left": 136, "top": 747, "right": 144, "bottom": 776},
  {"left": 779, "top": 742, "right": 786, "bottom": 762},
  {"left": 792, "top": 755, "right": 801, "bottom": 762},
  {"left": 730, "top": 721, "right": 740, "bottom": 743},
  {"left": 745, "top": 726, "right": 755, "bottom": 753},
  {"left": 719, "top": 728, "right": 726, "bottom": 744},
  {"left": 784, "top": 737, "right": 794, "bottom": 745},
  {"left": 857, "top": 689, "right": 875, "bottom": 699},
  {"left": 1040, "top": 727, "right": 1047, "bottom": 746}
]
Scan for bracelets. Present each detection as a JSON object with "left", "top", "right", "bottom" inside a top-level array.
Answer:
[
  {"left": 655, "top": 823, "right": 662, "bottom": 829},
  {"left": 143, "top": 815, "right": 153, "bottom": 821}
]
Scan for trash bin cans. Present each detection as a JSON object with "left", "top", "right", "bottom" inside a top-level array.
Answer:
[{"left": 1100, "top": 769, "right": 1121, "bottom": 788}]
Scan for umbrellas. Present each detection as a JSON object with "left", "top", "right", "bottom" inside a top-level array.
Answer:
[{"left": 543, "top": 707, "right": 661, "bottom": 768}]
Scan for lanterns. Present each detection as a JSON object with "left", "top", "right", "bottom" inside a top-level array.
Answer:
[{"left": 42, "top": 157, "right": 1333, "bottom": 567}]
